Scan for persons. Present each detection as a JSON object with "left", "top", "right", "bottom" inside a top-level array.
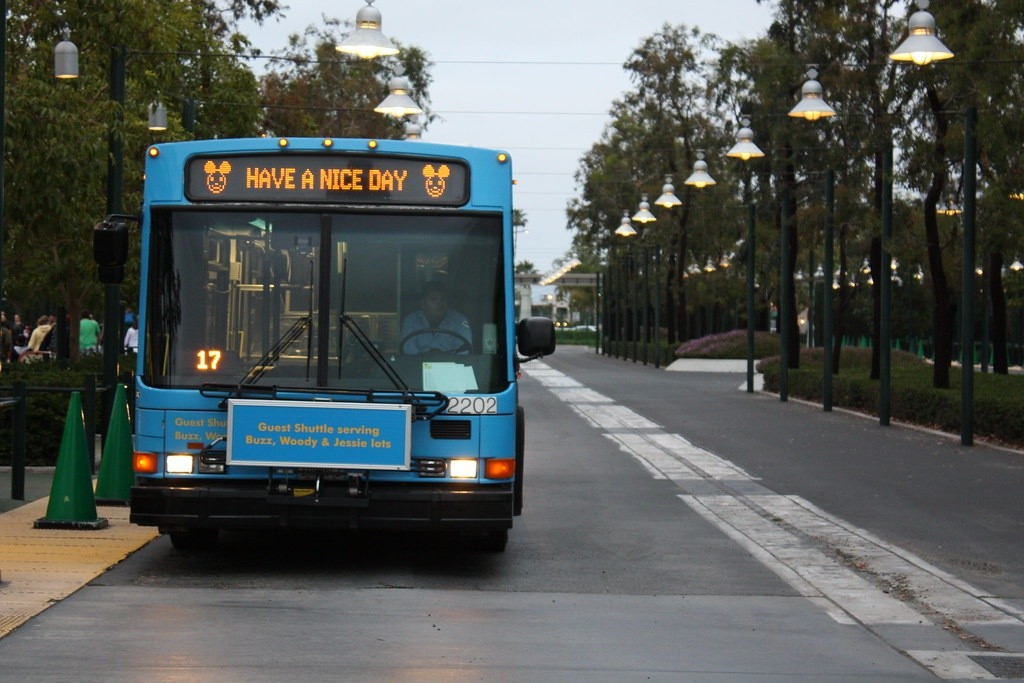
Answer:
[
  {"left": 401, "top": 283, "right": 472, "bottom": 354},
  {"left": 0, "top": 312, "right": 142, "bottom": 362}
]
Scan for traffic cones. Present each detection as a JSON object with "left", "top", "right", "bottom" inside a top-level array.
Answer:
[
  {"left": 34, "top": 392, "right": 112, "bottom": 531},
  {"left": 92, "top": 382, "right": 134, "bottom": 507}
]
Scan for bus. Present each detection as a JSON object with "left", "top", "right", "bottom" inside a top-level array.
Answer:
[{"left": 91, "top": 134, "right": 558, "bottom": 557}]
